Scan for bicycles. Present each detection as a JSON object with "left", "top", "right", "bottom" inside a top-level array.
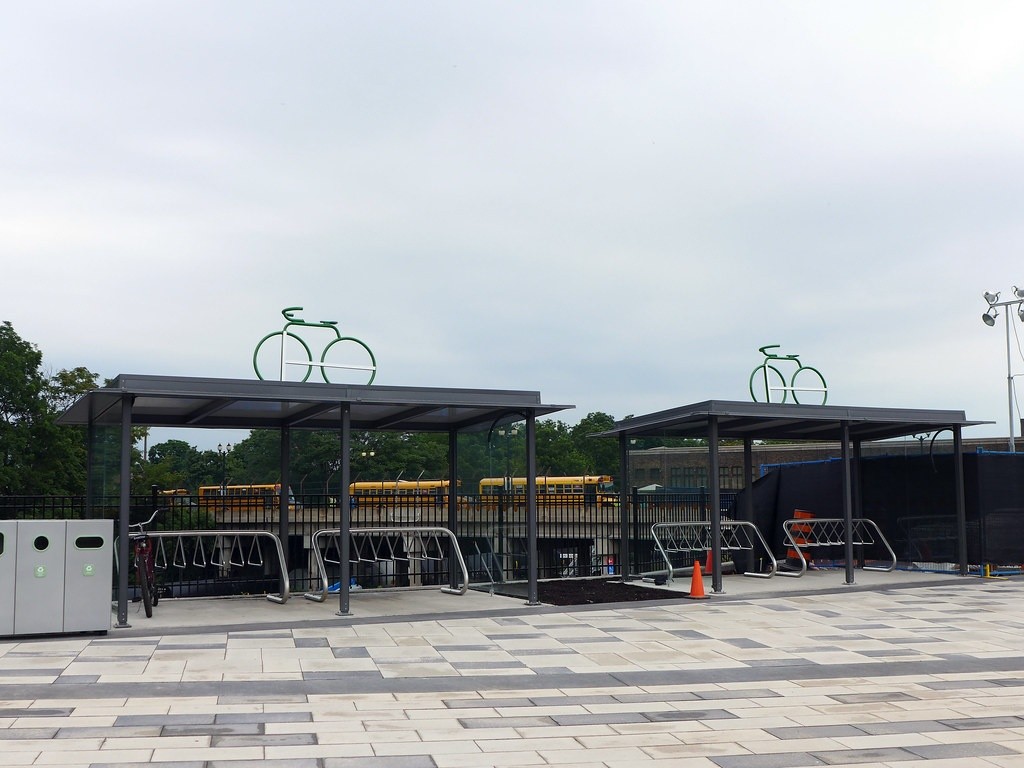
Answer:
[{"left": 118, "top": 509, "right": 159, "bottom": 619}]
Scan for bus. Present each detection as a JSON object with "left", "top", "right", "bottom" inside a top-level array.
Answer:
[
  {"left": 199, "top": 484, "right": 303, "bottom": 511},
  {"left": 349, "top": 480, "right": 470, "bottom": 510},
  {"left": 479, "top": 474, "right": 616, "bottom": 504}
]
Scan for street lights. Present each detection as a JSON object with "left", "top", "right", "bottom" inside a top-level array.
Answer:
[{"left": 218, "top": 444, "right": 232, "bottom": 579}]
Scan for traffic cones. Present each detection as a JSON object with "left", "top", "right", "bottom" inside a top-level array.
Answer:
[
  {"left": 702, "top": 547, "right": 714, "bottom": 575},
  {"left": 684, "top": 560, "right": 710, "bottom": 599}
]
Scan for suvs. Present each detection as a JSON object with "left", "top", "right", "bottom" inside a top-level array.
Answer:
[{"left": 158, "top": 488, "right": 197, "bottom": 512}]
